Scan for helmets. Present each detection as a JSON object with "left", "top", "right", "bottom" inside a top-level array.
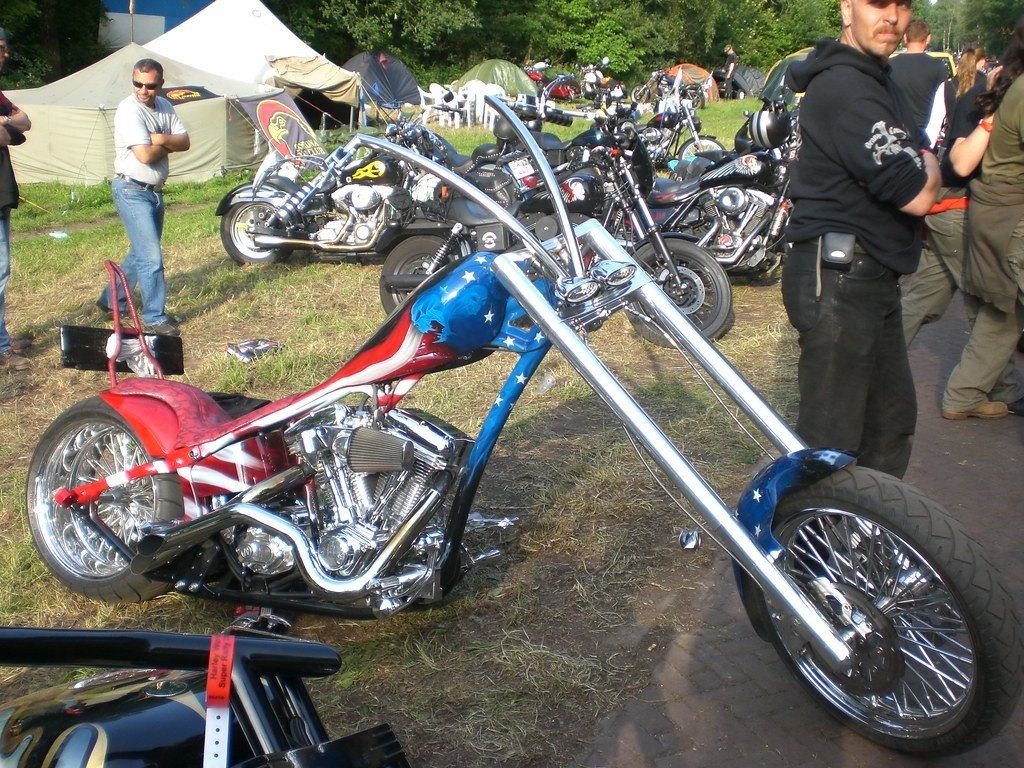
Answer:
[
  {"left": 748, "top": 110, "right": 792, "bottom": 150},
  {"left": 472, "top": 143, "right": 501, "bottom": 167},
  {"left": 494, "top": 116, "right": 514, "bottom": 140}
]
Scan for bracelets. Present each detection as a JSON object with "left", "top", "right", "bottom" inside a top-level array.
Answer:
[
  {"left": 920, "top": 149, "right": 930, "bottom": 154},
  {"left": 5, "top": 116, "right": 11, "bottom": 125}
]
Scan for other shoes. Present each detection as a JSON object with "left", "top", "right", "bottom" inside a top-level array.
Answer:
[
  {"left": 0, "top": 351, "right": 30, "bottom": 370},
  {"left": 1007, "top": 398, "right": 1024, "bottom": 417},
  {"left": 143, "top": 322, "right": 180, "bottom": 337},
  {"left": 96, "top": 303, "right": 129, "bottom": 320}
]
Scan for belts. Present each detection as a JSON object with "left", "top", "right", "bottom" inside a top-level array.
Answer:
[
  {"left": 811, "top": 237, "right": 867, "bottom": 254},
  {"left": 117, "top": 174, "right": 162, "bottom": 192}
]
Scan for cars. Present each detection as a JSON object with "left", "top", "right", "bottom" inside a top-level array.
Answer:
[{"left": 927, "top": 51, "right": 957, "bottom": 80}]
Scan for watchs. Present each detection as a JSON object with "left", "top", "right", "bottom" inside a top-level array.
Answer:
[{"left": 978, "top": 119, "right": 993, "bottom": 131}]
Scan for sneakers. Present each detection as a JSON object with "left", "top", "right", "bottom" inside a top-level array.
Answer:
[{"left": 942, "top": 401, "right": 1009, "bottom": 421}]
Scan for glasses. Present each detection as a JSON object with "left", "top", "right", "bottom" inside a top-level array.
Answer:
[
  {"left": 0, "top": 45, "right": 9, "bottom": 52},
  {"left": 132, "top": 79, "right": 161, "bottom": 90}
]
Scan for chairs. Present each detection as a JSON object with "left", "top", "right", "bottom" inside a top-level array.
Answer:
[{"left": 416, "top": 80, "right": 514, "bottom": 131}]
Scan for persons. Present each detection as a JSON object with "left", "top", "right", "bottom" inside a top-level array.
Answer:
[
  {"left": 95, "top": 60, "right": 191, "bottom": 340},
  {"left": 782, "top": 0, "right": 940, "bottom": 578},
  {"left": 722, "top": 44, "right": 739, "bottom": 101},
  {"left": 0, "top": 28, "right": 36, "bottom": 367},
  {"left": 887, "top": 18, "right": 1024, "bottom": 419}
]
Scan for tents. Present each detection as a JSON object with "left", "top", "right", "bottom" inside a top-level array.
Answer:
[
  {"left": 143, "top": 0, "right": 370, "bottom": 130},
  {"left": 759, "top": 47, "right": 815, "bottom": 107},
  {"left": 341, "top": 49, "right": 420, "bottom": 110},
  {"left": 713, "top": 66, "right": 765, "bottom": 98},
  {"left": 0, "top": 44, "right": 338, "bottom": 186},
  {"left": 450, "top": 59, "right": 538, "bottom": 97},
  {"left": 642, "top": 61, "right": 719, "bottom": 103}
]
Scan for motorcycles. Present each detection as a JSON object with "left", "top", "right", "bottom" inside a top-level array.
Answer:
[
  {"left": 23, "top": 93, "right": 1024, "bottom": 760},
  {"left": 214, "top": 48, "right": 813, "bottom": 351},
  {"left": 0, "top": 614, "right": 412, "bottom": 768}
]
